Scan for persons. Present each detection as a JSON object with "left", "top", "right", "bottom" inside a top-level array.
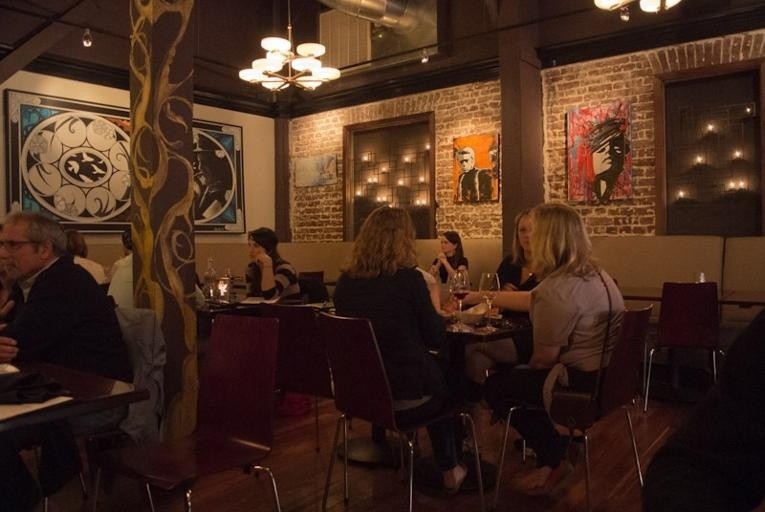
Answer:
[
  {"left": 107, "top": 228, "right": 133, "bottom": 282},
  {"left": 0, "top": 212, "right": 134, "bottom": 508},
  {"left": 331, "top": 204, "right": 469, "bottom": 494},
  {"left": 584, "top": 106, "right": 629, "bottom": 203},
  {"left": 429, "top": 227, "right": 469, "bottom": 288},
  {"left": 63, "top": 227, "right": 106, "bottom": 287},
  {"left": 455, "top": 147, "right": 494, "bottom": 203},
  {"left": 451, "top": 201, "right": 628, "bottom": 498},
  {"left": 244, "top": 226, "right": 303, "bottom": 299},
  {"left": 491, "top": 208, "right": 538, "bottom": 366},
  {"left": 0, "top": 265, "right": 24, "bottom": 335}
]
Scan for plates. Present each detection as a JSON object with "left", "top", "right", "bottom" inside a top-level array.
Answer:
[{"left": 454, "top": 302, "right": 489, "bottom": 323}]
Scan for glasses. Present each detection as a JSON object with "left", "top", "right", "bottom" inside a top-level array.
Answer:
[{"left": 0, "top": 239, "right": 29, "bottom": 253}]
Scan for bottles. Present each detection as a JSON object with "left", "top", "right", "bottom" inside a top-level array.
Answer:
[{"left": 204, "top": 254, "right": 234, "bottom": 303}]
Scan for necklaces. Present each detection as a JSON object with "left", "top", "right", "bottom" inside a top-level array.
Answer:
[{"left": 526, "top": 263, "right": 534, "bottom": 278}]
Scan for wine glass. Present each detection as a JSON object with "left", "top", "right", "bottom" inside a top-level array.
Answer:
[
  {"left": 479, "top": 272, "right": 500, "bottom": 332},
  {"left": 445, "top": 270, "right": 472, "bottom": 333}
]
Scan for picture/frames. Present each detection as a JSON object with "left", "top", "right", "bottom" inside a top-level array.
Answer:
[{"left": 3, "top": 87, "right": 247, "bottom": 235}]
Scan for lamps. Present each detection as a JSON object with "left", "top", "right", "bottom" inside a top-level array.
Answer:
[
  {"left": 594, "top": 0, "right": 682, "bottom": 21},
  {"left": 238, "top": 0, "right": 342, "bottom": 92}
]
